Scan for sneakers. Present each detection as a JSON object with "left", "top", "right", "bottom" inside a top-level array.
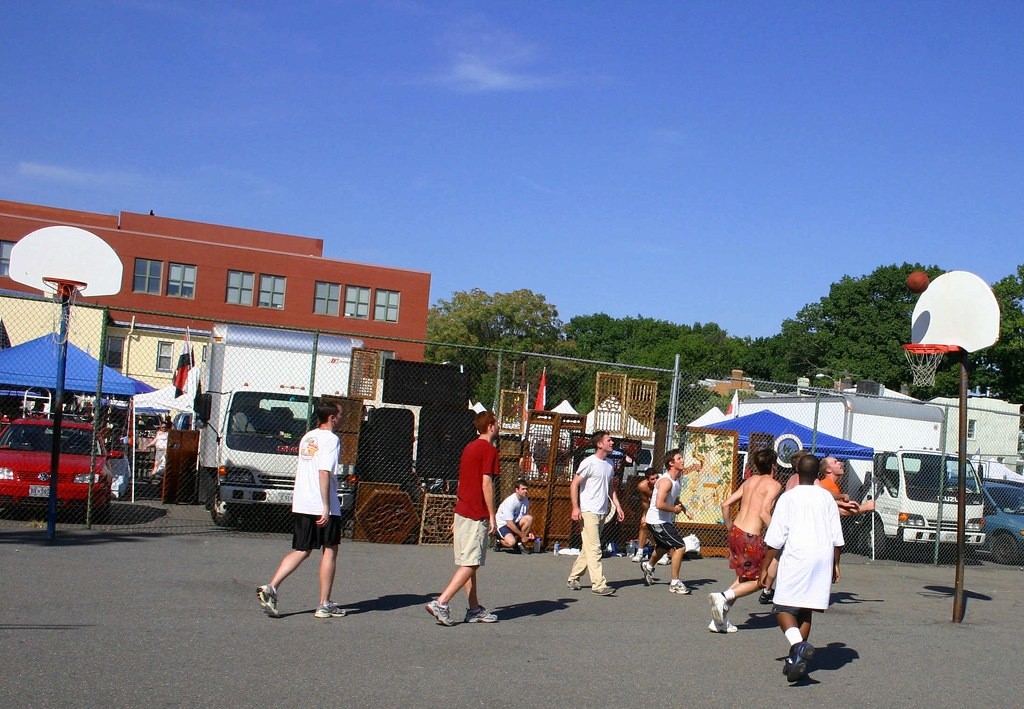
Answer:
[
  {"left": 640, "top": 562, "right": 656, "bottom": 585},
  {"left": 426, "top": 600, "right": 455, "bottom": 627},
  {"left": 256, "top": 585, "right": 282, "bottom": 618},
  {"left": 776, "top": 640, "right": 814, "bottom": 683},
  {"left": 632, "top": 552, "right": 643, "bottom": 562},
  {"left": 314, "top": 602, "right": 346, "bottom": 618},
  {"left": 465, "top": 606, "right": 497, "bottom": 623},
  {"left": 493, "top": 539, "right": 501, "bottom": 552},
  {"left": 758, "top": 588, "right": 775, "bottom": 603},
  {"left": 657, "top": 553, "right": 672, "bottom": 566},
  {"left": 708, "top": 592, "right": 729, "bottom": 626},
  {"left": 708, "top": 619, "right": 738, "bottom": 633},
  {"left": 513, "top": 542, "right": 531, "bottom": 555},
  {"left": 566, "top": 579, "right": 582, "bottom": 590},
  {"left": 669, "top": 581, "right": 691, "bottom": 595},
  {"left": 592, "top": 587, "right": 616, "bottom": 596}
]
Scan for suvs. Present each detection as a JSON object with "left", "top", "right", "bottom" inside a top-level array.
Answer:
[{"left": 946, "top": 477, "right": 1024, "bottom": 566}]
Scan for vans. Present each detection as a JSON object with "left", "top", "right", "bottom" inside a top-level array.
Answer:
[{"left": 635, "top": 447, "right": 654, "bottom": 475}]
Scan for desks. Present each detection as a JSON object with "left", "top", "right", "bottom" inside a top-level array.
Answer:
[{"left": 129, "top": 450, "right": 152, "bottom": 485}]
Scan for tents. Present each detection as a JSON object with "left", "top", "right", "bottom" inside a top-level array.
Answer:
[
  {"left": 0, "top": 331, "right": 158, "bottom": 503},
  {"left": 697, "top": 410, "right": 880, "bottom": 563}
]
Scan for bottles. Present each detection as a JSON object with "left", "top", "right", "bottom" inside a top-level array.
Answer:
[
  {"left": 626, "top": 540, "right": 635, "bottom": 557},
  {"left": 648, "top": 547, "right": 654, "bottom": 560},
  {"left": 533, "top": 538, "right": 541, "bottom": 552},
  {"left": 554, "top": 541, "right": 560, "bottom": 556}
]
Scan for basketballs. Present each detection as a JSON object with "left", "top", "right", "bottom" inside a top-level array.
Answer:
[{"left": 907, "top": 270, "right": 930, "bottom": 294}]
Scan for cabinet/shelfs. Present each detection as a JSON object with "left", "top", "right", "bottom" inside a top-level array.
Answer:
[{"left": 521, "top": 409, "right": 586, "bottom": 553}]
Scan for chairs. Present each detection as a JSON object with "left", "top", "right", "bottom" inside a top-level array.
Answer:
[
  {"left": 918, "top": 459, "right": 948, "bottom": 487},
  {"left": 10, "top": 432, "right": 40, "bottom": 450},
  {"left": 232, "top": 405, "right": 272, "bottom": 433},
  {"left": 62, "top": 434, "right": 93, "bottom": 455},
  {"left": 293, "top": 419, "right": 307, "bottom": 437}
]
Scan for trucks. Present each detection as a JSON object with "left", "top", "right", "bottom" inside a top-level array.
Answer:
[
  {"left": 738, "top": 394, "right": 986, "bottom": 558},
  {"left": 198, "top": 324, "right": 366, "bottom": 526}
]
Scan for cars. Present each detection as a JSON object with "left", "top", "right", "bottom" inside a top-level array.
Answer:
[{"left": 0, "top": 418, "right": 125, "bottom": 525}]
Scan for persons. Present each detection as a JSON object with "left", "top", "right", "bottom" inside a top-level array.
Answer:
[
  {"left": 631, "top": 467, "right": 673, "bottom": 566},
  {"left": 565, "top": 431, "right": 626, "bottom": 595},
  {"left": 254, "top": 397, "right": 348, "bottom": 619},
  {"left": 640, "top": 447, "right": 692, "bottom": 595},
  {"left": 1, "top": 393, "right": 187, "bottom": 479},
  {"left": 702, "top": 445, "right": 884, "bottom": 686},
  {"left": 492, "top": 480, "right": 535, "bottom": 554},
  {"left": 425, "top": 411, "right": 499, "bottom": 626}
]
